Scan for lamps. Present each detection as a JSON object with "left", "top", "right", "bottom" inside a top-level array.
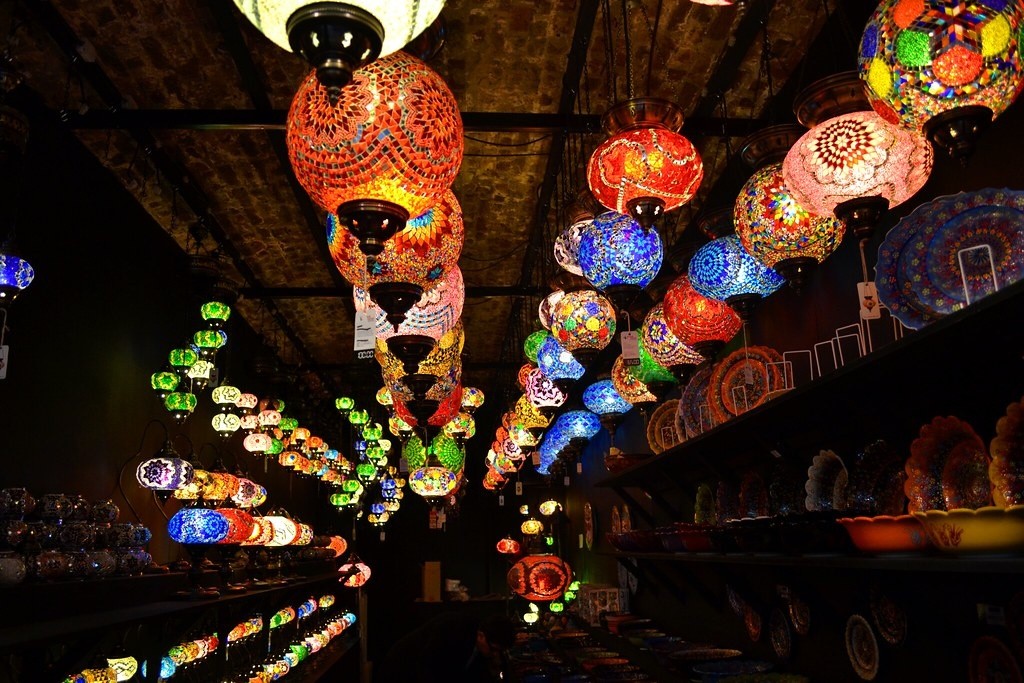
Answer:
[{"left": 117, "top": 0, "right": 1019, "bottom": 555}]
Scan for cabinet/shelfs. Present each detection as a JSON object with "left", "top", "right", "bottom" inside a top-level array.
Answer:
[
  {"left": 597, "top": 279, "right": 1019, "bottom": 602},
  {"left": 0, "top": 566, "right": 358, "bottom": 683}
]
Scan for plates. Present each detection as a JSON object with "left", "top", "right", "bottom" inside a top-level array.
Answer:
[
  {"left": 873, "top": 187, "right": 1024, "bottom": 330},
  {"left": 740, "top": 587, "right": 908, "bottom": 682},
  {"left": 646, "top": 345, "right": 794, "bottom": 456},
  {"left": 967, "top": 590, "right": 1024, "bottom": 683}
]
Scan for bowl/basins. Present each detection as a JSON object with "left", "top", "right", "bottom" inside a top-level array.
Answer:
[
  {"left": 504, "top": 610, "right": 811, "bottom": 683},
  {"left": 603, "top": 506, "right": 1024, "bottom": 553}
]
